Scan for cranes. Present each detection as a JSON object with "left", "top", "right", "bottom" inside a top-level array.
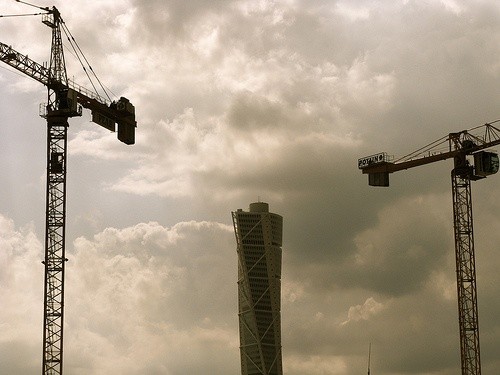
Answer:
[
  {"left": 0, "top": 0, "right": 138, "bottom": 375},
  {"left": 358, "top": 118, "right": 500, "bottom": 375}
]
